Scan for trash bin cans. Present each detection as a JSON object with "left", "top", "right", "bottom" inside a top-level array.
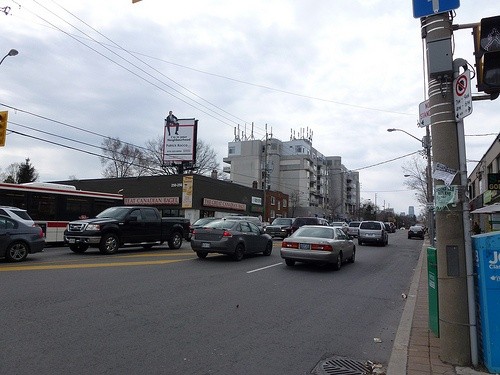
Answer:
[
  {"left": 471, "top": 231, "right": 500, "bottom": 374},
  {"left": 427, "top": 247, "right": 439, "bottom": 337}
]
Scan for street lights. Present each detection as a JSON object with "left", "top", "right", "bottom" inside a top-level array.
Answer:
[{"left": 386, "top": 128, "right": 435, "bottom": 247}]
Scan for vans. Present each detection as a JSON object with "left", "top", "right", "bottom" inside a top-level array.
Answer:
[{"left": 224, "top": 215, "right": 267, "bottom": 232}]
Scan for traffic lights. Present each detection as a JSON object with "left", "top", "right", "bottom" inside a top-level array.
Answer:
[
  {"left": 476, "top": 15, "right": 500, "bottom": 95},
  {"left": 0, "top": 111, "right": 9, "bottom": 148}
]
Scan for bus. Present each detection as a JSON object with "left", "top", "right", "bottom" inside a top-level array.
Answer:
[{"left": 0, "top": 181, "right": 126, "bottom": 248}]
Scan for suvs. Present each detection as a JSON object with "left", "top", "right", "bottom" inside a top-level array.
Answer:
[{"left": 1, "top": 206, "right": 36, "bottom": 227}]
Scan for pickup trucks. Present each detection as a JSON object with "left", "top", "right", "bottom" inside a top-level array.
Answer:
[{"left": 62, "top": 205, "right": 190, "bottom": 255}]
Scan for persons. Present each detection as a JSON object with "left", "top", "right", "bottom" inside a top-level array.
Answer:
[
  {"left": 342, "top": 225, "right": 349, "bottom": 235},
  {"left": 473, "top": 221, "right": 482, "bottom": 235},
  {"left": 167, "top": 111, "right": 180, "bottom": 136}
]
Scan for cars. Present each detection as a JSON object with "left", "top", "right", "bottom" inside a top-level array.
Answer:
[
  {"left": 190, "top": 220, "right": 274, "bottom": 262},
  {"left": 280, "top": 216, "right": 429, "bottom": 247},
  {"left": 358, "top": 220, "right": 388, "bottom": 246},
  {"left": 262, "top": 218, "right": 296, "bottom": 238},
  {"left": 0, "top": 214, "right": 45, "bottom": 263},
  {"left": 187, "top": 216, "right": 223, "bottom": 239},
  {"left": 279, "top": 224, "right": 357, "bottom": 271}
]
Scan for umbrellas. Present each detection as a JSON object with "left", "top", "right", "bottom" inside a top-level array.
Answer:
[{"left": 469, "top": 203, "right": 500, "bottom": 213}]
